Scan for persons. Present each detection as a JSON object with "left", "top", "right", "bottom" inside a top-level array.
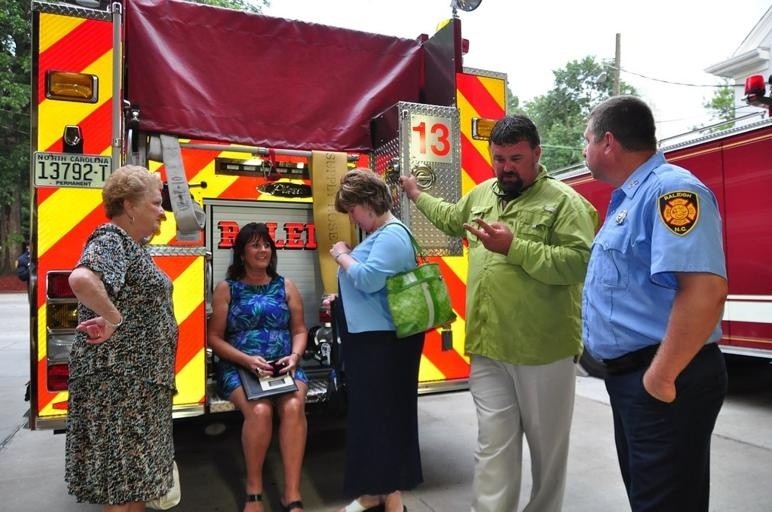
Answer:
[
  {"left": 64, "top": 164, "right": 179, "bottom": 512},
  {"left": 327, "top": 167, "right": 428, "bottom": 511},
  {"left": 398, "top": 114, "right": 600, "bottom": 511},
  {"left": 582, "top": 94, "right": 729, "bottom": 512},
  {"left": 206, "top": 221, "right": 312, "bottom": 512}
]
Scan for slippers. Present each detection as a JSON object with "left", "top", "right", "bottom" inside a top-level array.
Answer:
[
  {"left": 243, "top": 494, "right": 264, "bottom": 512},
  {"left": 344, "top": 499, "right": 384, "bottom": 512},
  {"left": 279, "top": 500, "right": 304, "bottom": 512}
]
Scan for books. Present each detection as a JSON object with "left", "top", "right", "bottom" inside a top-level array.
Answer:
[{"left": 237, "top": 358, "right": 299, "bottom": 400}]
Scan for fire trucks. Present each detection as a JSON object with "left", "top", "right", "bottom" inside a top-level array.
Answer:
[
  {"left": 534, "top": 73, "right": 772, "bottom": 377},
  {"left": 23, "top": 1, "right": 512, "bottom": 432}
]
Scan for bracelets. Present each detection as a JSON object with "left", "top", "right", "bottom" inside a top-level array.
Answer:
[
  {"left": 112, "top": 316, "right": 123, "bottom": 327},
  {"left": 334, "top": 251, "right": 348, "bottom": 262}
]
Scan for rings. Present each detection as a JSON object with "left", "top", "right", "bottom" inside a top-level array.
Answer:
[
  {"left": 97, "top": 333, "right": 100, "bottom": 336},
  {"left": 256, "top": 366, "right": 263, "bottom": 374}
]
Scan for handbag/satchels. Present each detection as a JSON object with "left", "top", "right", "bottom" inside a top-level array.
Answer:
[
  {"left": 379, "top": 222, "right": 457, "bottom": 340},
  {"left": 327, "top": 299, "right": 347, "bottom": 420},
  {"left": 145, "top": 461, "right": 181, "bottom": 511}
]
